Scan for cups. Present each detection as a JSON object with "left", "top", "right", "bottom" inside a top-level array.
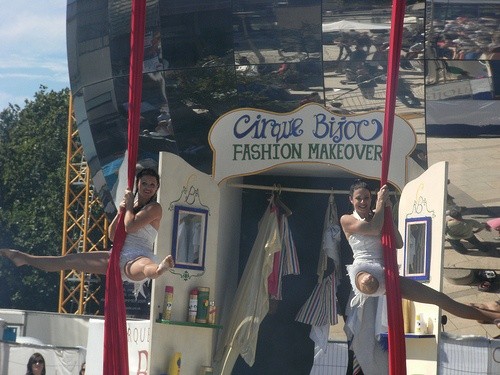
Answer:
[{"left": 196, "top": 287, "right": 210, "bottom": 324}]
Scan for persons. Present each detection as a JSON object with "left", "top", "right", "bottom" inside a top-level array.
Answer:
[
  {"left": 294, "top": 13, "right": 500, "bottom": 100},
  {"left": 78, "top": 359, "right": 86, "bottom": 375},
  {"left": 25, "top": 352, "right": 46, "bottom": 375},
  {"left": 142, "top": 31, "right": 262, "bottom": 117},
  {"left": 0, "top": 167, "right": 175, "bottom": 284},
  {"left": 339, "top": 177, "right": 500, "bottom": 325},
  {"left": 440, "top": 179, "right": 500, "bottom": 339}
]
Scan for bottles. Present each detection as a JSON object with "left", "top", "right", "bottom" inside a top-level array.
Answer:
[
  {"left": 207, "top": 301, "right": 216, "bottom": 323},
  {"left": 188, "top": 290, "right": 198, "bottom": 322},
  {"left": 162, "top": 285, "right": 173, "bottom": 321}
]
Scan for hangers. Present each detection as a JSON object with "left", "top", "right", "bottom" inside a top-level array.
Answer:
[
  {"left": 268, "top": 184, "right": 292, "bottom": 215},
  {"left": 330, "top": 187, "right": 335, "bottom": 204}
]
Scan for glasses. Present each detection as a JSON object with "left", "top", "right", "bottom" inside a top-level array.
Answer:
[{"left": 31, "top": 360, "right": 44, "bottom": 364}]
